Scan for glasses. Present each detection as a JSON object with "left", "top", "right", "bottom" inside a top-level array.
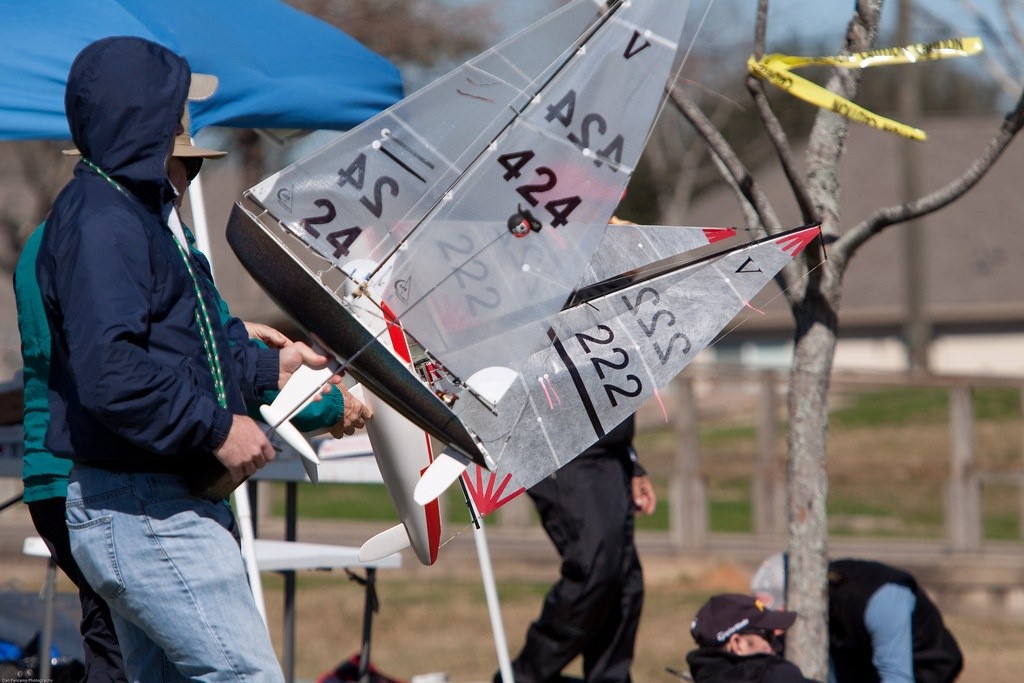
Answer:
[{"left": 179, "top": 157, "right": 204, "bottom": 181}]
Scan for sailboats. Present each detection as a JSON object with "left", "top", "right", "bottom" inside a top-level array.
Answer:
[
  {"left": 359, "top": 215, "right": 824, "bottom": 558},
  {"left": 237, "top": 0, "right": 693, "bottom": 468}
]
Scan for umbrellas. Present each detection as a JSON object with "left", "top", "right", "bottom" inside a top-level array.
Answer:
[{"left": 0, "top": 0, "right": 407, "bottom": 143}]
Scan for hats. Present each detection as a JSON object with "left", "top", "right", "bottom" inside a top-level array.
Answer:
[
  {"left": 175, "top": 107, "right": 228, "bottom": 162},
  {"left": 688, "top": 594, "right": 798, "bottom": 650},
  {"left": 186, "top": 71, "right": 217, "bottom": 100},
  {"left": 746, "top": 553, "right": 788, "bottom": 612}
]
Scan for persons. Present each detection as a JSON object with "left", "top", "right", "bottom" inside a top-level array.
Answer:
[
  {"left": 687, "top": 591, "right": 826, "bottom": 682},
  {"left": 35, "top": 33, "right": 343, "bottom": 683},
  {"left": 488, "top": 213, "right": 659, "bottom": 682},
  {"left": 13, "top": 97, "right": 376, "bottom": 682},
  {"left": 750, "top": 550, "right": 967, "bottom": 683}
]
